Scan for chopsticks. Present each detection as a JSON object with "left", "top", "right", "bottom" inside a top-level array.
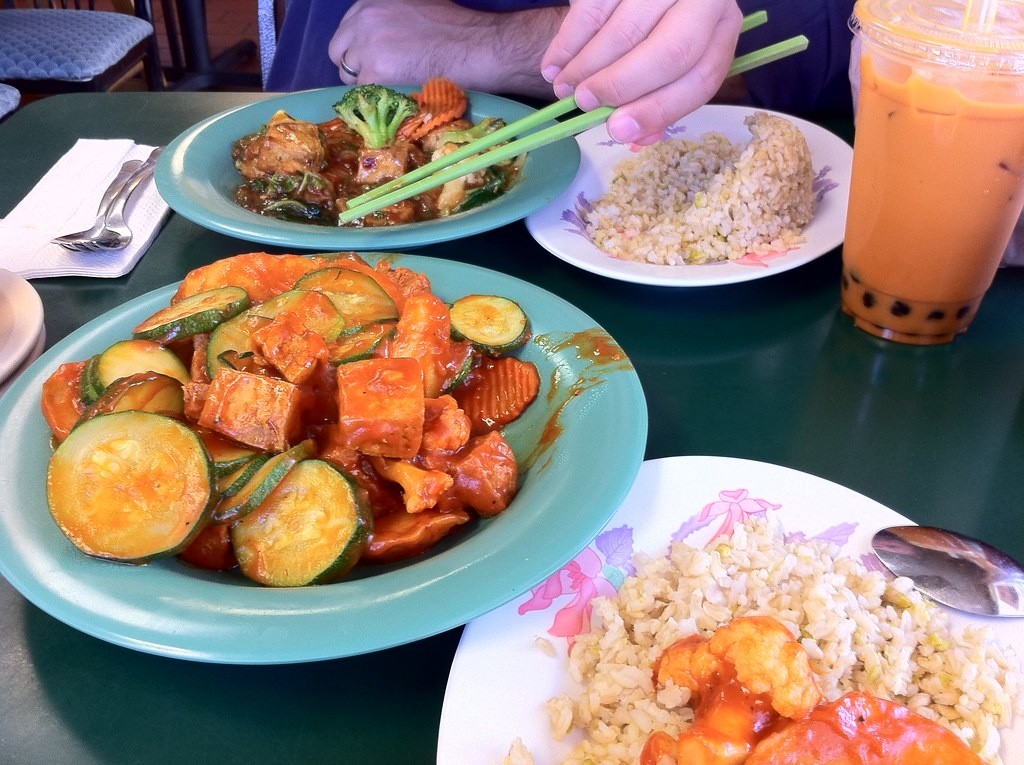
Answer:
[{"left": 338, "top": 9, "right": 809, "bottom": 226}]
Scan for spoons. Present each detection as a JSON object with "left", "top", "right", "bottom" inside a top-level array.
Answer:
[
  {"left": 52, "top": 160, "right": 147, "bottom": 244},
  {"left": 871, "top": 525, "right": 1024, "bottom": 621}
]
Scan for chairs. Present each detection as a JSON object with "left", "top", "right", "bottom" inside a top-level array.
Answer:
[{"left": 1, "top": 0, "right": 165, "bottom": 97}]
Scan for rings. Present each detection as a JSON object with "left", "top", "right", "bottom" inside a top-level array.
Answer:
[{"left": 340, "top": 50, "right": 362, "bottom": 78}]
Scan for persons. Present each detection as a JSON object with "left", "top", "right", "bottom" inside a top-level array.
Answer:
[{"left": 263, "top": 0, "right": 1024, "bottom": 272}]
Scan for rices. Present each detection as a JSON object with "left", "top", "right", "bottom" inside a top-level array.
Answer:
[
  {"left": 537, "top": 515, "right": 1023, "bottom": 765},
  {"left": 585, "top": 111, "right": 814, "bottom": 266}
]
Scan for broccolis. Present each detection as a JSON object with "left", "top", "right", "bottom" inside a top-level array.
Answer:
[{"left": 332, "top": 82, "right": 419, "bottom": 149}]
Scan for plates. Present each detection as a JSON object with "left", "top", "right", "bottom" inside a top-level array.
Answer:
[
  {"left": 153, "top": 85, "right": 581, "bottom": 251},
  {"left": 0, "top": 268, "right": 47, "bottom": 397},
  {"left": 437, "top": 454, "right": 1024, "bottom": 763},
  {"left": 523, "top": 104, "right": 854, "bottom": 288},
  {"left": 0, "top": 252, "right": 648, "bottom": 667}
]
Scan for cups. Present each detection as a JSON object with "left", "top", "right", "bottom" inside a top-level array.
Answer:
[{"left": 839, "top": 0, "right": 1024, "bottom": 345}]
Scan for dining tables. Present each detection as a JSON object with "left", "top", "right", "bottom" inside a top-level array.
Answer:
[{"left": 0, "top": 88, "right": 1024, "bottom": 764}]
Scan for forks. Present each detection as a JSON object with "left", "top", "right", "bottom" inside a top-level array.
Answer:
[{"left": 59, "top": 146, "right": 167, "bottom": 253}]
